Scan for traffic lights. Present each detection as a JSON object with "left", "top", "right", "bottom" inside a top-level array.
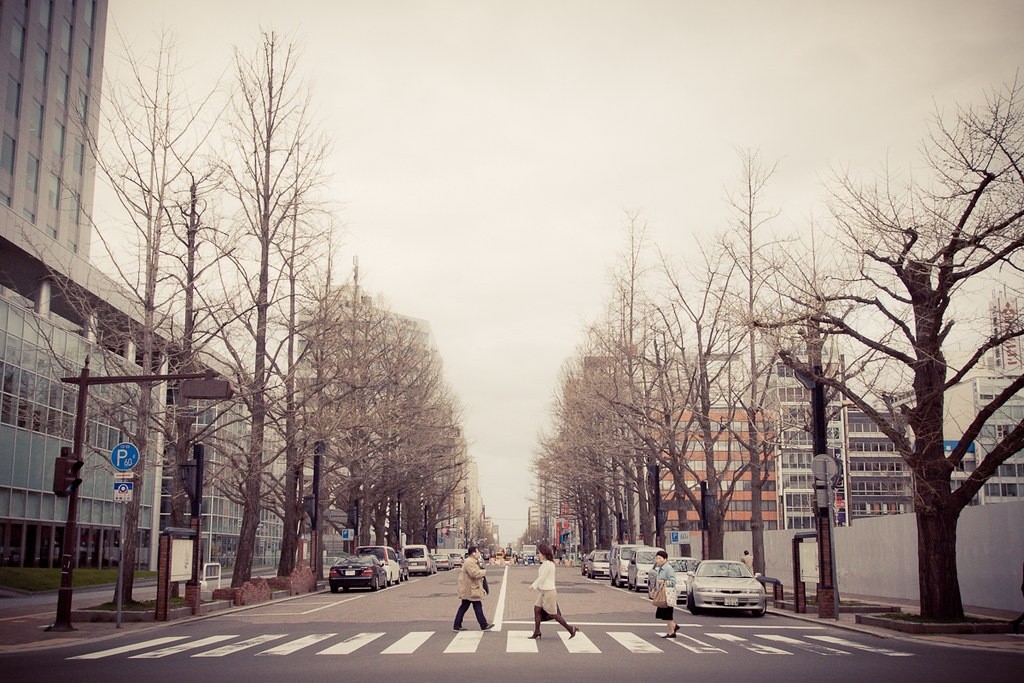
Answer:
[{"left": 53, "top": 457, "right": 84, "bottom": 496}]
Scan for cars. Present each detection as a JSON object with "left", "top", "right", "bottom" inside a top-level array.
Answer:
[
  {"left": 329, "top": 546, "right": 409, "bottom": 592},
  {"left": 647, "top": 557, "right": 702, "bottom": 600},
  {"left": 433, "top": 554, "right": 454, "bottom": 570},
  {"left": 581, "top": 545, "right": 649, "bottom": 588},
  {"left": 451, "top": 553, "right": 490, "bottom": 569},
  {"left": 685, "top": 560, "right": 766, "bottom": 617}
]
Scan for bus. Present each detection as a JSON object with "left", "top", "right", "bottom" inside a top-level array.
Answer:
[{"left": 496, "top": 545, "right": 537, "bottom": 565}]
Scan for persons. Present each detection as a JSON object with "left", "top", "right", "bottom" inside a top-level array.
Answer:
[
  {"left": 488, "top": 551, "right": 587, "bottom": 567},
  {"left": 655, "top": 550, "right": 680, "bottom": 638},
  {"left": 452, "top": 547, "right": 495, "bottom": 632},
  {"left": 741, "top": 549, "right": 753, "bottom": 573},
  {"left": 526, "top": 543, "right": 580, "bottom": 640}
]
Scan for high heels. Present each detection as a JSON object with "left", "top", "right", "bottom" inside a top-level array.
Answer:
[
  {"left": 528, "top": 632, "right": 542, "bottom": 639},
  {"left": 568, "top": 626, "right": 580, "bottom": 639}
]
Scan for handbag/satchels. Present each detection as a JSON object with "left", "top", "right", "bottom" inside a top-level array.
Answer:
[
  {"left": 482, "top": 576, "right": 489, "bottom": 599},
  {"left": 652, "top": 581, "right": 676, "bottom": 608},
  {"left": 540, "top": 603, "right": 561, "bottom": 622}
]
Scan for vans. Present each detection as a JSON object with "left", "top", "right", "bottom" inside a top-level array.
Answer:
[
  {"left": 628, "top": 547, "right": 666, "bottom": 591},
  {"left": 401, "top": 545, "right": 437, "bottom": 575}
]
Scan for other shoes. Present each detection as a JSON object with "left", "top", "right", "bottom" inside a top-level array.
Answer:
[
  {"left": 661, "top": 633, "right": 676, "bottom": 638},
  {"left": 454, "top": 628, "right": 468, "bottom": 631},
  {"left": 673, "top": 624, "right": 680, "bottom": 633},
  {"left": 482, "top": 623, "right": 495, "bottom": 630}
]
